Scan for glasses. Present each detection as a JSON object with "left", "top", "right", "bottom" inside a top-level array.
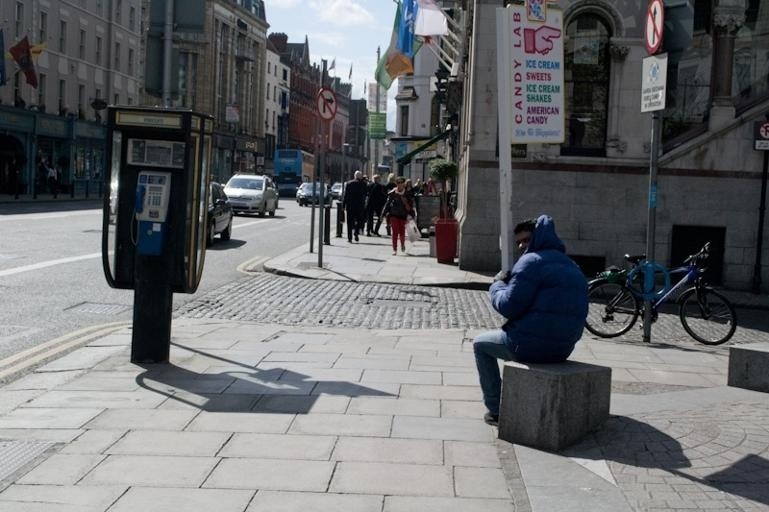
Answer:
[
  {"left": 396, "top": 181, "right": 406, "bottom": 184},
  {"left": 515, "top": 236, "right": 533, "bottom": 247}
]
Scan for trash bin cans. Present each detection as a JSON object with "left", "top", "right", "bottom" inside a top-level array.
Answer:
[{"left": 429, "top": 226, "right": 436, "bottom": 257}]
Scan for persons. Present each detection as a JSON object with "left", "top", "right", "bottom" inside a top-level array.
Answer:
[
  {"left": 472, "top": 215, "right": 588, "bottom": 426},
  {"left": 341, "top": 170, "right": 437, "bottom": 256}
]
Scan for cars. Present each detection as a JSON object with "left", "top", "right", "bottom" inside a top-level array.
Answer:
[
  {"left": 219, "top": 171, "right": 278, "bottom": 218},
  {"left": 293, "top": 179, "right": 347, "bottom": 208},
  {"left": 204, "top": 179, "right": 234, "bottom": 246}
]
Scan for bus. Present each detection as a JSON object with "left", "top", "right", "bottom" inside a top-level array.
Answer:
[
  {"left": 271, "top": 147, "right": 317, "bottom": 196},
  {"left": 370, "top": 165, "right": 392, "bottom": 175}
]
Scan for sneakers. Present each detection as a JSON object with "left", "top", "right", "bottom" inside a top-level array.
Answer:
[
  {"left": 387, "top": 232, "right": 405, "bottom": 255},
  {"left": 483, "top": 410, "right": 500, "bottom": 427},
  {"left": 347, "top": 230, "right": 381, "bottom": 242}
]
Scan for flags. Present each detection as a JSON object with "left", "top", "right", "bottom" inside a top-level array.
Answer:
[
  {"left": 375, "top": 0, "right": 449, "bottom": 90},
  {"left": 6, "top": 34, "right": 39, "bottom": 90}
]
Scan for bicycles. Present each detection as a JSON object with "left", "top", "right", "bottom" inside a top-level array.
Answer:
[{"left": 584, "top": 238, "right": 738, "bottom": 348}]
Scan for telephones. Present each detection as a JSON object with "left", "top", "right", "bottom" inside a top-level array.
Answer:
[{"left": 135, "top": 171, "right": 172, "bottom": 222}]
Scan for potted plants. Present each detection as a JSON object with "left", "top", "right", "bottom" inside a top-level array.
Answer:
[{"left": 426, "top": 156, "right": 461, "bottom": 265}]
